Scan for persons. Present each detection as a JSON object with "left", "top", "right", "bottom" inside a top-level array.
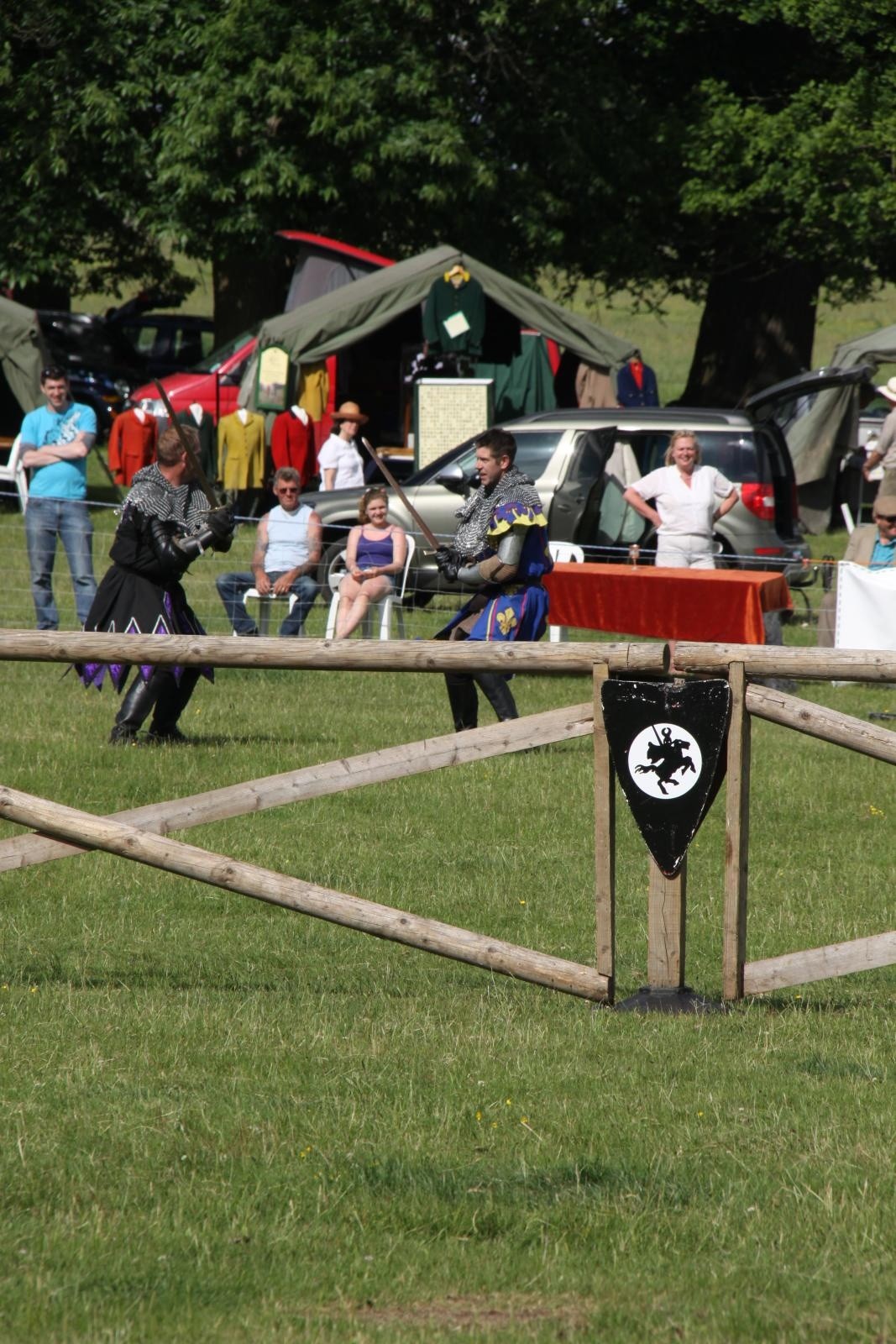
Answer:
[
  {"left": 334, "top": 486, "right": 406, "bottom": 639},
  {"left": 318, "top": 401, "right": 368, "bottom": 493},
  {"left": 432, "top": 429, "right": 555, "bottom": 732},
  {"left": 862, "top": 376, "right": 896, "bottom": 496},
  {"left": 817, "top": 495, "right": 896, "bottom": 648},
  {"left": 73, "top": 423, "right": 237, "bottom": 747},
  {"left": 17, "top": 366, "right": 99, "bottom": 631},
  {"left": 622, "top": 430, "right": 740, "bottom": 570},
  {"left": 216, "top": 467, "right": 322, "bottom": 638}
]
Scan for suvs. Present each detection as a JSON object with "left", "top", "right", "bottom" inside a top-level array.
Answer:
[
  {"left": 35, "top": 307, "right": 220, "bottom": 440},
  {"left": 283, "top": 363, "right": 881, "bottom": 617}
]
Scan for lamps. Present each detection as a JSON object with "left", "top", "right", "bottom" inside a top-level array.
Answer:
[{"left": 631, "top": 353, "right": 638, "bottom": 363}]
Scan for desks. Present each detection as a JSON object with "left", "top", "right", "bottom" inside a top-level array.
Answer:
[
  {"left": 835, "top": 442, "right": 875, "bottom": 528},
  {"left": 541, "top": 562, "right": 797, "bottom": 690}
]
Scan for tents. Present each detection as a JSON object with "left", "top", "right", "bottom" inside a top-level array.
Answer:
[
  {"left": 782, "top": 321, "right": 895, "bottom": 535},
  {"left": 237, "top": 245, "right": 642, "bottom": 527}
]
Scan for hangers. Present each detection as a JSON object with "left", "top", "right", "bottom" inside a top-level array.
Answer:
[{"left": 449, "top": 261, "right": 465, "bottom": 274}]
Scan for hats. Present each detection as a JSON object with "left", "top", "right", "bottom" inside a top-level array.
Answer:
[
  {"left": 328, "top": 402, "right": 368, "bottom": 426},
  {"left": 877, "top": 376, "right": 896, "bottom": 402},
  {"left": 874, "top": 496, "right": 896, "bottom": 517}
]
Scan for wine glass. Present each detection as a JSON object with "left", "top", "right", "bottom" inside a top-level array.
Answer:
[{"left": 629, "top": 544, "right": 640, "bottom": 570}]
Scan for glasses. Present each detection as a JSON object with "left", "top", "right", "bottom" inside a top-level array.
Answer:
[
  {"left": 43, "top": 369, "right": 60, "bottom": 378},
  {"left": 276, "top": 488, "right": 297, "bottom": 494}
]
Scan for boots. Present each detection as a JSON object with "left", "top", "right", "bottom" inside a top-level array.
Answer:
[
  {"left": 109, "top": 673, "right": 166, "bottom": 745},
  {"left": 148, "top": 667, "right": 201, "bottom": 742}
]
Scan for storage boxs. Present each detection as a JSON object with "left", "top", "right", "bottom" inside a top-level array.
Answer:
[{"left": 850, "top": 416, "right": 884, "bottom": 452}]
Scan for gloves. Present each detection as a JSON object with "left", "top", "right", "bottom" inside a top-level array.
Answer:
[
  {"left": 208, "top": 508, "right": 235, "bottom": 553},
  {"left": 433, "top": 547, "right": 463, "bottom": 583}
]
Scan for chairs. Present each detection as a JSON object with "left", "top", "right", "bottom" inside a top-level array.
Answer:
[
  {"left": 0, "top": 433, "right": 28, "bottom": 514},
  {"left": 233, "top": 588, "right": 305, "bottom": 637},
  {"left": 325, "top": 533, "right": 416, "bottom": 639},
  {"left": 602, "top": 441, "right": 645, "bottom": 542},
  {"left": 547, "top": 540, "right": 585, "bottom": 642}
]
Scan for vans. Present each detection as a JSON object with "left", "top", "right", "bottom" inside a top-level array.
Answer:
[{"left": 105, "top": 227, "right": 562, "bottom": 496}]
